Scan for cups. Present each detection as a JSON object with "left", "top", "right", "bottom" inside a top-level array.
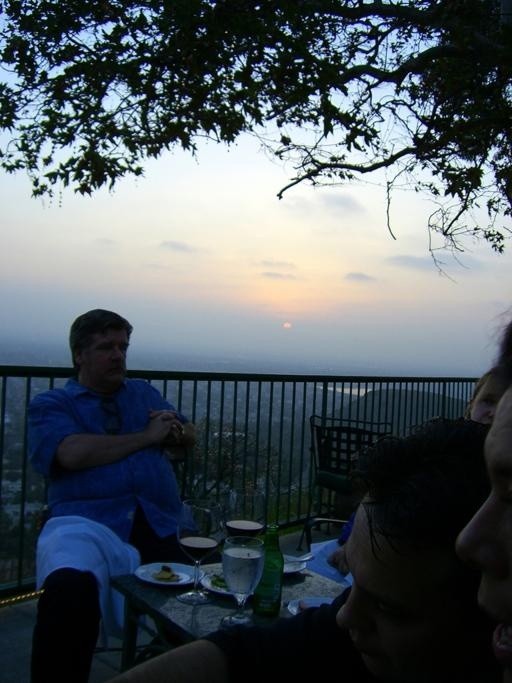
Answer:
[{"left": 222, "top": 487, "right": 268, "bottom": 547}]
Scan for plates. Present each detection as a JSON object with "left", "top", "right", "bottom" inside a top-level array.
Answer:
[
  {"left": 200, "top": 567, "right": 258, "bottom": 596},
  {"left": 281, "top": 552, "right": 307, "bottom": 575},
  {"left": 286, "top": 596, "right": 336, "bottom": 616},
  {"left": 134, "top": 561, "right": 206, "bottom": 587}
]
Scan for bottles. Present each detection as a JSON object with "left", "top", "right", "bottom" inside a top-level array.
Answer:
[{"left": 252, "top": 522, "right": 284, "bottom": 619}]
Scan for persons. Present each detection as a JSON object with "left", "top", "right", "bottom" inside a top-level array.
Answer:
[
  {"left": 451, "top": 382, "right": 511, "bottom": 682},
  {"left": 326, "top": 506, "right": 361, "bottom": 576},
  {"left": 109, "top": 412, "right": 500, "bottom": 683},
  {"left": 24, "top": 306, "right": 208, "bottom": 682},
  {"left": 462, "top": 364, "right": 511, "bottom": 426}
]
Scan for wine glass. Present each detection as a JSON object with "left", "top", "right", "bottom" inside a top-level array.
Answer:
[
  {"left": 219, "top": 535, "right": 266, "bottom": 630},
  {"left": 175, "top": 498, "right": 226, "bottom": 606}
]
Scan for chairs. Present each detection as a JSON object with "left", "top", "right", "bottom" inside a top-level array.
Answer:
[{"left": 292, "top": 413, "right": 394, "bottom": 549}]
